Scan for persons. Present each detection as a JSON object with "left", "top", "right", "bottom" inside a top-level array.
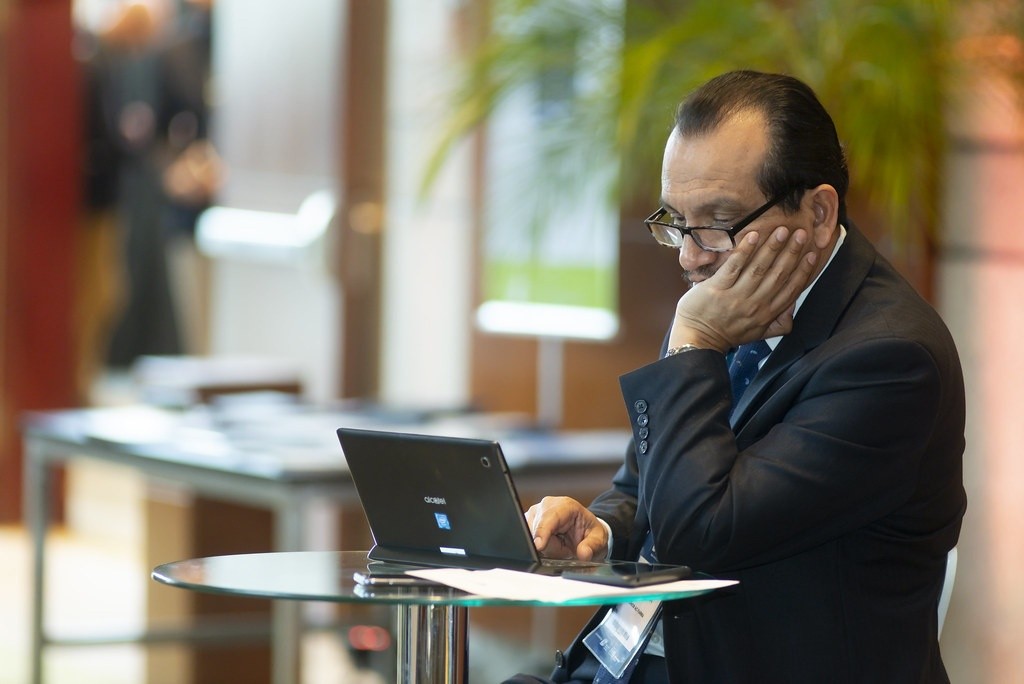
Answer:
[{"left": 500, "top": 70, "right": 968, "bottom": 683}]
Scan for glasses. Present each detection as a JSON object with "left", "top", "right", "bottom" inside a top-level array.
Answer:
[{"left": 643, "top": 184, "right": 813, "bottom": 252}]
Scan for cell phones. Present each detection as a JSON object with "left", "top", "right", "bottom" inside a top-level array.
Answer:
[{"left": 561, "top": 561, "right": 692, "bottom": 587}]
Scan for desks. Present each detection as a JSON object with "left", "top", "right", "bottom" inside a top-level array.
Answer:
[
  {"left": 153, "top": 553, "right": 742, "bottom": 684},
  {"left": 25, "top": 410, "right": 638, "bottom": 684}
]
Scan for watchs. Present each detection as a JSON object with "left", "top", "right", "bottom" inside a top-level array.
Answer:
[{"left": 665, "top": 344, "right": 698, "bottom": 358}]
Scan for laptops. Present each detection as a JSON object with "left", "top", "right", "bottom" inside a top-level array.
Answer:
[{"left": 335, "top": 427, "right": 625, "bottom": 576}]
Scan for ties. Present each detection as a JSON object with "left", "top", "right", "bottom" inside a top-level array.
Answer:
[{"left": 592, "top": 339, "right": 773, "bottom": 684}]
